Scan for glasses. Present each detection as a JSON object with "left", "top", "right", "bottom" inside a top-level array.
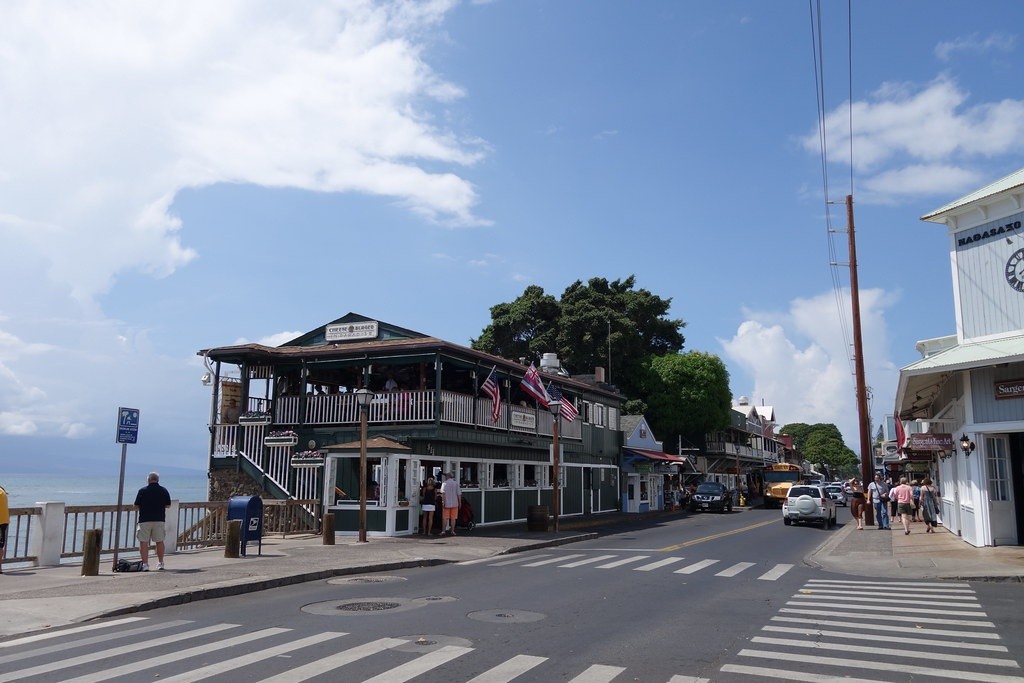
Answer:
[{"left": 854, "top": 484, "right": 859, "bottom": 485}]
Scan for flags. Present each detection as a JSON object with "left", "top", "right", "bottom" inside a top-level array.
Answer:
[
  {"left": 518, "top": 362, "right": 552, "bottom": 409},
  {"left": 481, "top": 366, "right": 501, "bottom": 424},
  {"left": 545, "top": 382, "right": 580, "bottom": 422}
]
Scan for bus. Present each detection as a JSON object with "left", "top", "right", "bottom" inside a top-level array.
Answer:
[{"left": 762, "top": 463, "right": 805, "bottom": 509}]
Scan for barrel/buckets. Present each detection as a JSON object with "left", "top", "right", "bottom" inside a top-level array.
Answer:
[{"left": 527, "top": 505, "right": 548, "bottom": 532}]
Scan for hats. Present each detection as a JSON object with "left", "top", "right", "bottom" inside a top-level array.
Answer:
[
  {"left": 445, "top": 472, "right": 454, "bottom": 477},
  {"left": 886, "top": 480, "right": 890, "bottom": 483}
]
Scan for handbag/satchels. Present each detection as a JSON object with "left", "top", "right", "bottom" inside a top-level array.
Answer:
[
  {"left": 878, "top": 495, "right": 890, "bottom": 503},
  {"left": 116, "top": 559, "right": 143, "bottom": 572},
  {"left": 918, "top": 507, "right": 924, "bottom": 520},
  {"left": 935, "top": 505, "right": 940, "bottom": 514},
  {"left": 910, "top": 499, "right": 916, "bottom": 509}
]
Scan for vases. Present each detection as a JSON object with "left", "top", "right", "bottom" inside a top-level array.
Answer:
[
  {"left": 291, "top": 458, "right": 324, "bottom": 464},
  {"left": 264, "top": 437, "right": 298, "bottom": 445},
  {"left": 239, "top": 417, "right": 272, "bottom": 426}
]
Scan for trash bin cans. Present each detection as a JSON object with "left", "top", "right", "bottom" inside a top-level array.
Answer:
[
  {"left": 227, "top": 496, "right": 264, "bottom": 555},
  {"left": 527, "top": 504, "right": 549, "bottom": 532}
]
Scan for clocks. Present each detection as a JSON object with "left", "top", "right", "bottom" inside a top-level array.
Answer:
[{"left": 1005, "top": 248, "right": 1024, "bottom": 292}]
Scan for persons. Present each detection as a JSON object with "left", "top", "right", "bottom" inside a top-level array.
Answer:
[
  {"left": 420, "top": 470, "right": 442, "bottom": 535},
  {"left": 133, "top": 472, "right": 171, "bottom": 571},
  {"left": 849, "top": 478, "right": 867, "bottom": 530},
  {"left": 502, "top": 397, "right": 533, "bottom": 410},
  {"left": 867, "top": 474, "right": 892, "bottom": 530},
  {"left": 886, "top": 477, "right": 940, "bottom": 535},
  {"left": 0, "top": 486, "right": 10, "bottom": 574},
  {"left": 440, "top": 473, "right": 462, "bottom": 536},
  {"left": 278, "top": 374, "right": 414, "bottom": 420},
  {"left": 741, "top": 481, "right": 748, "bottom": 503}
]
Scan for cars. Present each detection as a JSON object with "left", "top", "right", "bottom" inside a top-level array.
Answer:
[
  {"left": 809, "top": 480, "right": 853, "bottom": 507},
  {"left": 691, "top": 481, "right": 732, "bottom": 514}
]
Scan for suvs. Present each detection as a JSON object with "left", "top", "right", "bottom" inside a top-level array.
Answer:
[{"left": 782, "top": 485, "right": 837, "bottom": 530}]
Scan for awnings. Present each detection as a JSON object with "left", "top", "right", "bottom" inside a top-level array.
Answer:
[{"left": 628, "top": 448, "right": 686, "bottom": 463}]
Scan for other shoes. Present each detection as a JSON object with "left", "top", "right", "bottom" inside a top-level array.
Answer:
[
  {"left": 929, "top": 530, "right": 934, "bottom": 534},
  {"left": 926, "top": 528, "right": 930, "bottom": 532},
  {"left": 438, "top": 532, "right": 445, "bottom": 536},
  {"left": 156, "top": 562, "right": 165, "bottom": 570},
  {"left": 905, "top": 531, "right": 910, "bottom": 535},
  {"left": 878, "top": 527, "right": 883, "bottom": 530},
  {"left": 450, "top": 532, "right": 457, "bottom": 536},
  {"left": 856, "top": 526, "right": 863, "bottom": 530},
  {"left": 142, "top": 564, "right": 149, "bottom": 571},
  {"left": 884, "top": 527, "right": 892, "bottom": 530}
]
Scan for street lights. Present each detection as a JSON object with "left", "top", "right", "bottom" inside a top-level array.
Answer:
[
  {"left": 547, "top": 397, "right": 564, "bottom": 534},
  {"left": 355, "top": 385, "right": 374, "bottom": 542}
]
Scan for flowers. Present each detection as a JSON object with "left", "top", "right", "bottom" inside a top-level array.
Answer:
[
  {"left": 269, "top": 430, "right": 299, "bottom": 437},
  {"left": 242, "top": 409, "right": 274, "bottom": 417},
  {"left": 292, "top": 451, "right": 323, "bottom": 459}
]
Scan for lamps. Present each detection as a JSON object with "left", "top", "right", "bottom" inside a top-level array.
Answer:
[
  {"left": 912, "top": 402, "right": 919, "bottom": 412},
  {"left": 1005, "top": 228, "right": 1024, "bottom": 244},
  {"left": 916, "top": 394, "right": 923, "bottom": 401},
  {"left": 938, "top": 449, "right": 956, "bottom": 463},
  {"left": 960, "top": 432, "right": 975, "bottom": 456}
]
